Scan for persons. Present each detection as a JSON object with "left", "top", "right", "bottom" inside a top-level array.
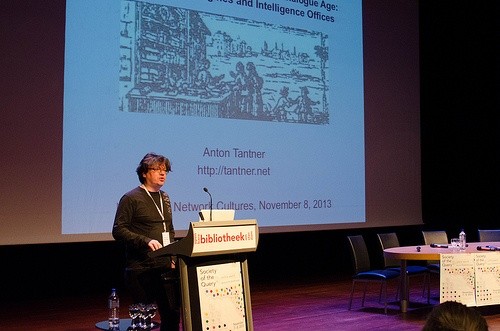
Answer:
[
  {"left": 112, "top": 153, "right": 180, "bottom": 331},
  {"left": 421, "top": 301, "right": 488, "bottom": 331}
]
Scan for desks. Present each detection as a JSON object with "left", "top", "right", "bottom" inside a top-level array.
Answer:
[{"left": 383, "top": 242, "right": 500, "bottom": 313}]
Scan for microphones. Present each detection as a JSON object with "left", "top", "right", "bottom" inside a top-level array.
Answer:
[
  {"left": 430, "top": 243, "right": 448, "bottom": 248},
  {"left": 477, "top": 246, "right": 496, "bottom": 251},
  {"left": 416, "top": 247, "right": 421, "bottom": 252},
  {"left": 203, "top": 187, "right": 212, "bottom": 221}
]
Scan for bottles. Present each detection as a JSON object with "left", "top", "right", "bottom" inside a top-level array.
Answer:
[
  {"left": 109, "top": 288, "right": 120, "bottom": 330},
  {"left": 459, "top": 229, "right": 466, "bottom": 252}
]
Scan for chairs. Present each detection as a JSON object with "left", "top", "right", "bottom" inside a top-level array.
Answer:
[
  {"left": 421, "top": 230, "right": 449, "bottom": 305},
  {"left": 479, "top": 229, "right": 500, "bottom": 242},
  {"left": 346, "top": 235, "right": 402, "bottom": 313},
  {"left": 377, "top": 232, "right": 430, "bottom": 306}
]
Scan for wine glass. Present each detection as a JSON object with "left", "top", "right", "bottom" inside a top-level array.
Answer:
[{"left": 126, "top": 303, "right": 159, "bottom": 331}]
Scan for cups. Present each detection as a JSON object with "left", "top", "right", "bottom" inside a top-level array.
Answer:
[{"left": 451, "top": 239, "right": 460, "bottom": 248}]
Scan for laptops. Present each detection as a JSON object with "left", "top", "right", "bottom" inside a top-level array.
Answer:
[{"left": 199, "top": 209, "right": 237, "bottom": 222}]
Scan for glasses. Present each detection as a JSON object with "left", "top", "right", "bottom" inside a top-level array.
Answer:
[{"left": 148, "top": 165, "right": 168, "bottom": 172}]
